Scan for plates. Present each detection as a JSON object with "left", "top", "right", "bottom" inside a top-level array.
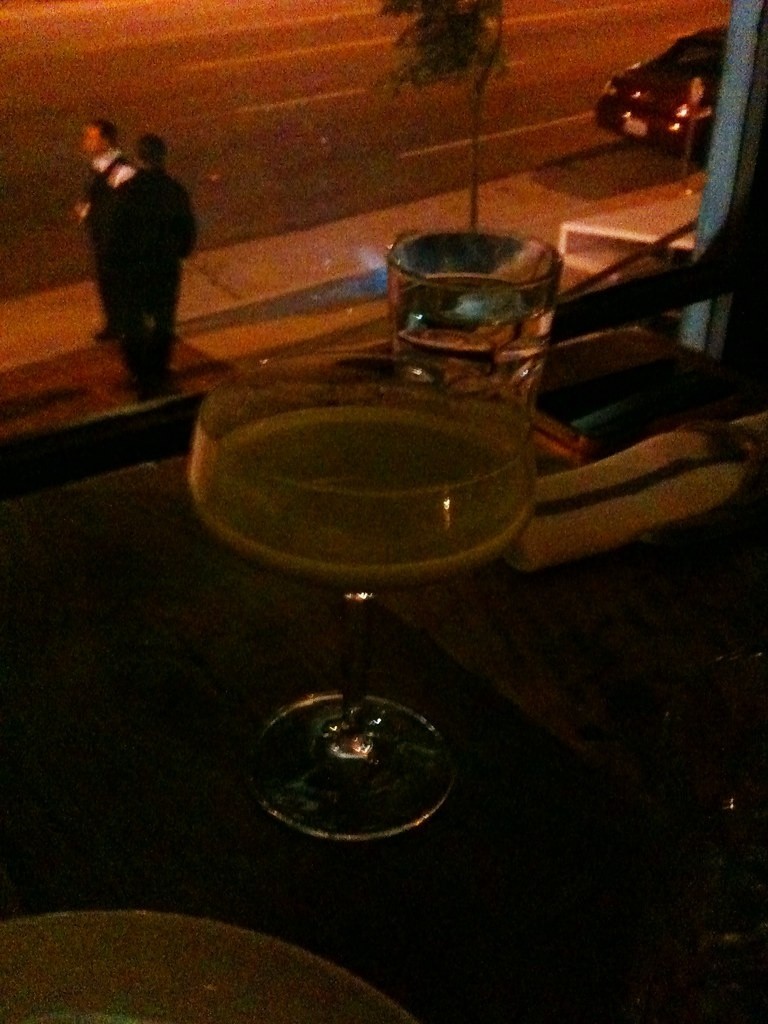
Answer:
[{"left": 0, "top": 911, "right": 419, "bottom": 1024}]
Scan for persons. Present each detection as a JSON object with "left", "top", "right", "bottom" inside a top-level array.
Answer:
[{"left": 78, "top": 120, "right": 197, "bottom": 403}]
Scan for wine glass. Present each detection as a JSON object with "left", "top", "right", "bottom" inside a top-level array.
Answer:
[{"left": 182, "top": 351, "right": 540, "bottom": 841}]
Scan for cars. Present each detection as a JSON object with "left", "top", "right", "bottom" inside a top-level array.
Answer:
[{"left": 593, "top": 21, "right": 730, "bottom": 162}]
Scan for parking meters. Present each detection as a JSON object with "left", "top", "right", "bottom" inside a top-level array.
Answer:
[{"left": 678, "top": 76, "right": 706, "bottom": 188}]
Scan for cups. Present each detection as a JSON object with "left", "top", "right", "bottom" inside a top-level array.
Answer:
[{"left": 389, "top": 228, "right": 561, "bottom": 431}]
